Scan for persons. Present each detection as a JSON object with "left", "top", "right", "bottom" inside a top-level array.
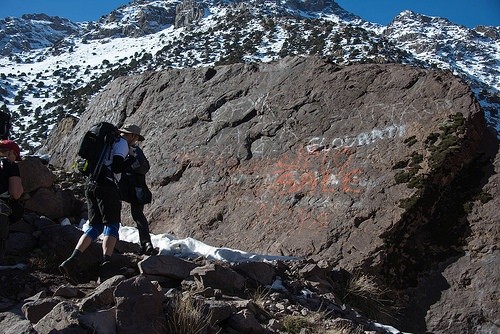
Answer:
[
  {"left": 59, "top": 123, "right": 158, "bottom": 286},
  {"left": 0, "top": 138, "right": 24, "bottom": 258}
]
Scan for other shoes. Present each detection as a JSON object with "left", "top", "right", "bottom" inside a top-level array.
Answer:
[
  {"left": 97, "top": 257, "right": 112, "bottom": 274},
  {"left": 59, "top": 260, "right": 79, "bottom": 286},
  {"left": 141, "top": 243, "right": 153, "bottom": 255}
]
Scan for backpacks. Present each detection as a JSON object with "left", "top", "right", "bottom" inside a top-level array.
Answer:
[{"left": 76, "top": 121, "right": 128, "bottom": 184}]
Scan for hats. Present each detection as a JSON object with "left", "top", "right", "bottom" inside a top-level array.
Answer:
[
  {"left": 118, "top": 124, "right": 145, "bottom": 142},
  {"left": 2, "top": 140, "right": 21, "bottom": 159}
]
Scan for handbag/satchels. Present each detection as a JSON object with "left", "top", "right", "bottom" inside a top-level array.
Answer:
[{"left": 0, "top": 197, "right": 25, "bottom": 224}]
